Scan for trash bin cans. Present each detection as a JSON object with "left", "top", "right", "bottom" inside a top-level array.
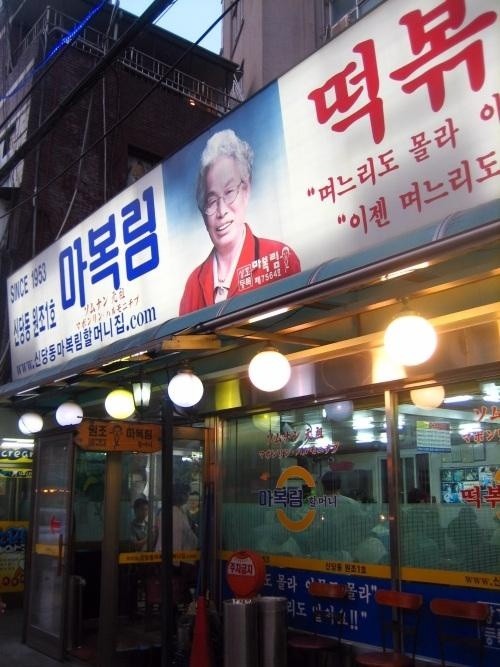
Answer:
[
  {"left": 259, "top": 596, "right": 288, "bottom": 667},
  {"left": 224, "top": 599, "right": 258, "bottom": 667}
]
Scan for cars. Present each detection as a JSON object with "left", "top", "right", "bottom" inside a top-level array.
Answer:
[{"left": 30, "top": 504, "right": 69, "bottom": 601}]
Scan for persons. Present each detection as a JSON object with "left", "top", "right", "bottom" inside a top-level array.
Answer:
[
  {"left": 179, "top": 129, "right": 301, "bottom": 317},
  {"left": 128, "top": 468, "right": 149, "bottom": 520},
  {"left": 312, "top": 471, "right": 500, "bottom": 574},
  {"left": 128, "top": 482, "right": 201, "bottom": 603}
]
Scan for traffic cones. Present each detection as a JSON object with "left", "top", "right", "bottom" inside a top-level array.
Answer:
[{"left": 188, "top": 595, "right": 217, "bottom": 666}]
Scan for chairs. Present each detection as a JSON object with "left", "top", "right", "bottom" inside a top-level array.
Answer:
[
  {"left": 288, "top": 583, "right": 353, "bottom": 664},
  {"left": 428, "top": 597, "right": 493, "bottom": 665},
  {"left": 355, "top": 588, "right": 425, "bottom": 667}
]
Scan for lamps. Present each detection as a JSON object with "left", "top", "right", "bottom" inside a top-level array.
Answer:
[
  {"left": 248, "top": 336, "right": 295, "bottom": 393},
  {"left": 381, "top": 286, "right": 439, "bottom": 368},
  {"left": 131, "top": 365, "right": 154, "bottom": 409},
  {"left": 166, "top": 358, "right": 205, "bottom": 407},
  {"left": 53, "top": 394, "right": 85, "bottom": 425},
  {"left": 104, "top": 380, "right": 136, "bottom": 418},
  {"left": 249, "top": 385, "right": 445, "bottom": 433},
  {"left": 18, "top": 409, "right": 44, "bottom": 433}
]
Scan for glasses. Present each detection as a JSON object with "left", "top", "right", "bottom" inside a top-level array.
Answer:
[{"left": 204, "top": 181, "right": 242, "bottom": 215}]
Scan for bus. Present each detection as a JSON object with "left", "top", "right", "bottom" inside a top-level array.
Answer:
[{"left": 0, "top": 436, "right": 145, "bottom": 616}]
